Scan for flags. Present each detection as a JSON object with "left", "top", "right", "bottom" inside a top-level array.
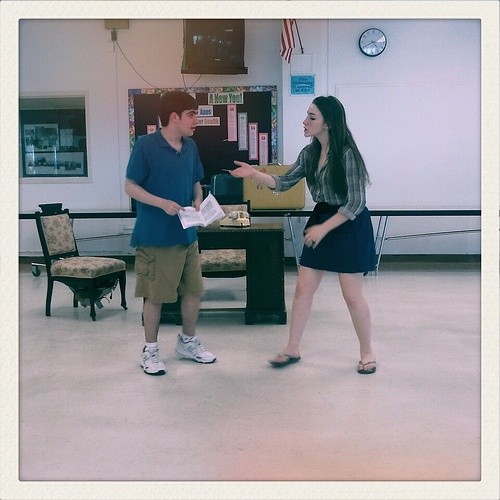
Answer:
[{"left": 279, "top": 19, "right": 295, "bottom": 62}]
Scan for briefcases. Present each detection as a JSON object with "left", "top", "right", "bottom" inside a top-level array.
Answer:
[
  {"left": 242, "top": 163, "right": 306, "bottom": 211},
  {"left": 211, "top": 170, "right": 243, "bottom": 205}
]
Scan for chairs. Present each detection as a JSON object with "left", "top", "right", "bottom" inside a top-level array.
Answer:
[
  {"left": 199, "top": 197, "right": 254, "bottom": 280},
  {"left": 33, "top": 200, "right": 130, "bottom": 322}
]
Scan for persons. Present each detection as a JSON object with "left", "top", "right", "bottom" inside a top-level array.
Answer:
[
  {"left": 228, "top": 95, "right": 377, "bottom": 374},
  {"left": 124, "top": 92, "right": 216, "bottom": 376}
]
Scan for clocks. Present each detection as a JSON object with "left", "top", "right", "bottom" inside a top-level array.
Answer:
[{"left": 358, "top": 27, "right": 388, "bottom": 57}]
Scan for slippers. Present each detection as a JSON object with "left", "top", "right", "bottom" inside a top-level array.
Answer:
[
  {"left": 357, "top": 361, "right": 376, "bottom": 375},
  {"left": 268, "top": 353, "right": 302, "bottom": 368}
]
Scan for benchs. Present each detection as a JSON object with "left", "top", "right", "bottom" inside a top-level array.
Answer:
[{"left": 20, "top": 203, "right": 481, "bottom": 278}]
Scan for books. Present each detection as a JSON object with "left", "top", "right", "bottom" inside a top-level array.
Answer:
[{"left": 177, "top": 194, "right": 226, "bottom": 229}]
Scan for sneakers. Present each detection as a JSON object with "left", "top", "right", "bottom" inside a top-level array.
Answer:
[
  {"left": 140, "top": 346, "right": 166, "bottom": 376},
  {"left": 174, "top": 333, "right": 217, "bottom": 364}
]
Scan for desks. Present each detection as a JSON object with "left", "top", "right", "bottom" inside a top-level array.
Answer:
[{"left": 141, "top": 222, "right": 289, "bottom": 326}]
